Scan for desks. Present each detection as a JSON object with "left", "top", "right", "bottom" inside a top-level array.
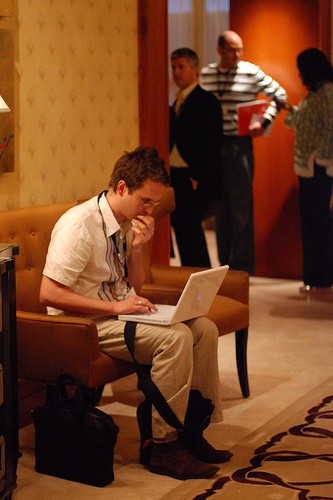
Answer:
[{"left": 0, "top": 242, "right": 22, "bottom": 488}]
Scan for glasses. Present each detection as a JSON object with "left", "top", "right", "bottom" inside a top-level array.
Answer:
[{"left": 133, "top": 191, "right": 160, "bottom": 209}]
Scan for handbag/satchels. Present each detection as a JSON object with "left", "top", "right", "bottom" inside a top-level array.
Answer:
[{"left": 30, "top": 373, "right": 119, "bottom": 488}]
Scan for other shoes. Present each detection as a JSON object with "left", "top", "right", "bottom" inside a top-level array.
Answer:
[
  {"left": 147, "top": 444, "right": 220, "bottom": 479},
  {"left": 176, "top": 427, "right": 233, "bottom": 464},
  {"left": 299, "top": 286, "right": 331, "bottom": 295}
]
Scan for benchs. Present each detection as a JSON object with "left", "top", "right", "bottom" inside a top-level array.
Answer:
[{"left": 0, "top": 196, "right": 251, "bottom": 406}]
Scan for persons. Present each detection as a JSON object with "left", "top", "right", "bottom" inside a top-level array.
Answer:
[
  {"left": 273, "top": 47, "right": 333, "bottom": 293},
  {"left": 168, "top": 48, "right": 224, "bottom": 268},
  {"left": 38, "top": 144, "right": 233, "bottom": 480},
  {"left": 198, "top": 30, "right": 288, "bottom": 273}
]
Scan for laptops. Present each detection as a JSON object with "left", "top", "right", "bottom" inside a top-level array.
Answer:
[{"left": 118, "top": 266, "right": 230, "bottom": 326}]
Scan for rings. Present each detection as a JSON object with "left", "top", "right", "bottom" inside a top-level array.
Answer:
[{"left": 137, "top": 300, "right": 141, "bottom": 305}]
